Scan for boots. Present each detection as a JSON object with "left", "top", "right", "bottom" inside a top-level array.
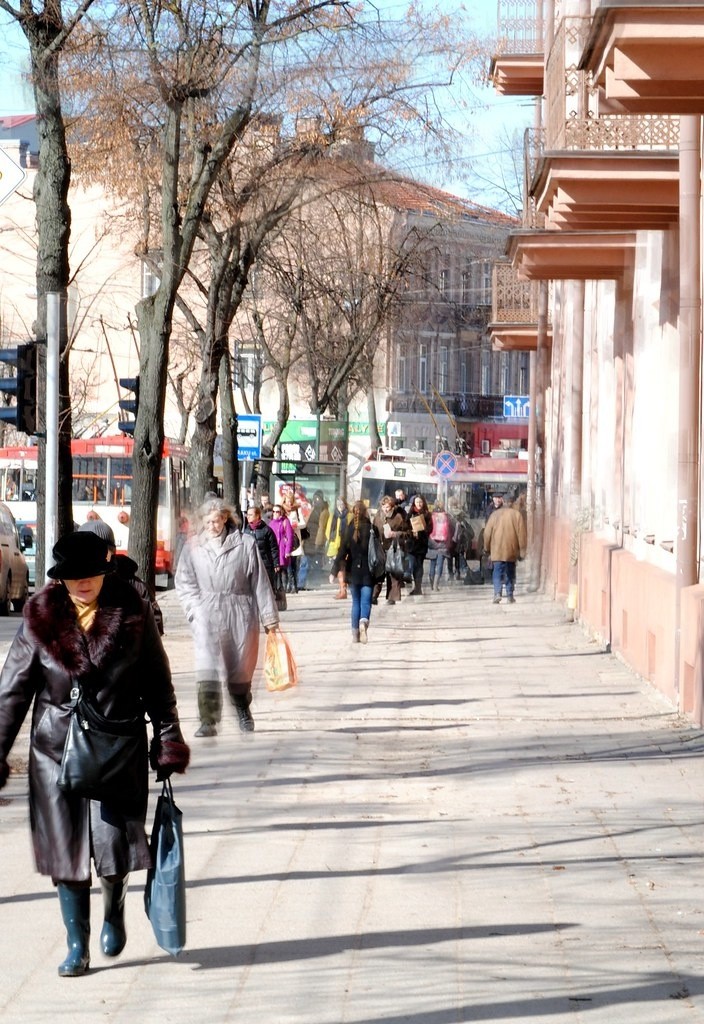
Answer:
[
  {"left": 359, "top": 617, "right": 369, "bottom": 644},
  {"left": 57, "top": 882, "right": 90, "bottom": 977},
  {"left": 352, "top": 628, "right": 360, "bottom": 643},
  {"left": 286, "top": 576, "right": 298, "bottom": 594},
  {"left": 100, "top": 871, "right": 129, "bottom": 956},
  {"left": 334, "top": 570, "right": 347, "bottom": 599}
]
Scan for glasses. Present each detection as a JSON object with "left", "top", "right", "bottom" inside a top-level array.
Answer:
[{"left": 272, "top": 511, "right": 281, "bottom": 514}]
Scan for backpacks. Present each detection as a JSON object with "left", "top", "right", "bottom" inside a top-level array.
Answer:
[{"left": 450, "top": 513, "right": 465, "bottom": 543}]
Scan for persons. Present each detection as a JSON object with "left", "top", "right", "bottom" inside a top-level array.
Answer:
[
  {"left": 0, "top": 519, "right": 190, "bottom": 975},
  {"left": 244, "top": 487, "right": 330, "bottom": 596},
  {"left": 325, "top": 494, "right": 356, "bottom": 600},
  {"left": 79, "top": 478, "right": 132, "bottom": 502},
  {"left": 373, "top": 488, "right": 475, "bottom": 604},
  {"left": 329, "top": 499, "right": 387, "bottom": 644},
  {"left": 483, "top": 488, "right": 527, "bottom": 604},
  {"left": 174, "top": 491, "right": 280, "bottom": 737}
]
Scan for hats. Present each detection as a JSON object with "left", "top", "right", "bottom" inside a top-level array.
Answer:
[
  {"left": 492, "top": 493, "right": 502, "bottom": 497},
  {"left": 77, "top": 519, "right": 116, "bottom": 553},
  {"left": 48, "top": 530, "right": 114, "bottom": 580},
  {"left": 502, "top": 493, "right": 515, "bottom": 501}
]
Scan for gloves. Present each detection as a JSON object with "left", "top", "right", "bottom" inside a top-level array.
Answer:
[{"left": 156, "top": 763, "right": 176, "bottom": 782}]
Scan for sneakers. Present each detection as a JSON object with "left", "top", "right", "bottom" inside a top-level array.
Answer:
[
  {"left": 195, "top": 720, "right": 217, "bottom": 737},
  {"left": 236, "top": 706, "right": 254, "bottom": 732}
]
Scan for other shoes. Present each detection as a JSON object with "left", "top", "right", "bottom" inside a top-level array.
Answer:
[
  {"left": 370, "top": 573, "right": 461, "bottom": 605},
  {"left": 508, "top": 595, "right": 515, "bottom": 603},
  {"left": 493, "top": 593, "right": 501, "bottom": 604}
]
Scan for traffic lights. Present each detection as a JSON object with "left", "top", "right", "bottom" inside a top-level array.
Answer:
[
  {"left": 118, "top": 377, "right": 142, "bottom": 438},
  {"left": 0, "top": 344, "right": 37, "bottom": 436}
]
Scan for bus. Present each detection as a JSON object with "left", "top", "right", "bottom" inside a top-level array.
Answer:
[
  {"left": 354, "top": 443, "right": 535, "bottom": 561},
  {"left": 0, "top": 435, "right": 193, "bottom": 586}
]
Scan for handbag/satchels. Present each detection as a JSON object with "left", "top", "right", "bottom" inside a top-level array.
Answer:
[
  {"left": 57, "top": 695, "right": 148, "bottom": 804},
  {"left": 282, "top": 517, "right": 300, "bottom": 551},
  {"left": 296, "top": 509, "right": 310, "bottom": 539},
  {"left": 367, "top": 522, "right": 385, "bottom": 578},
  {"left": 265, "top": 627, "right": 297, "bottom": 692},
  {"left": 272, "top": 570, "right": 287, "bottom": 611},
  {"left": 144, "top": 777, "right": 186, "bottom": 957},
  {"left": 409, "top": 512, "right": 427, "bottom": 539},
  {"left": 386, "top": 531, "right": 404, "bottom": 575}
]
